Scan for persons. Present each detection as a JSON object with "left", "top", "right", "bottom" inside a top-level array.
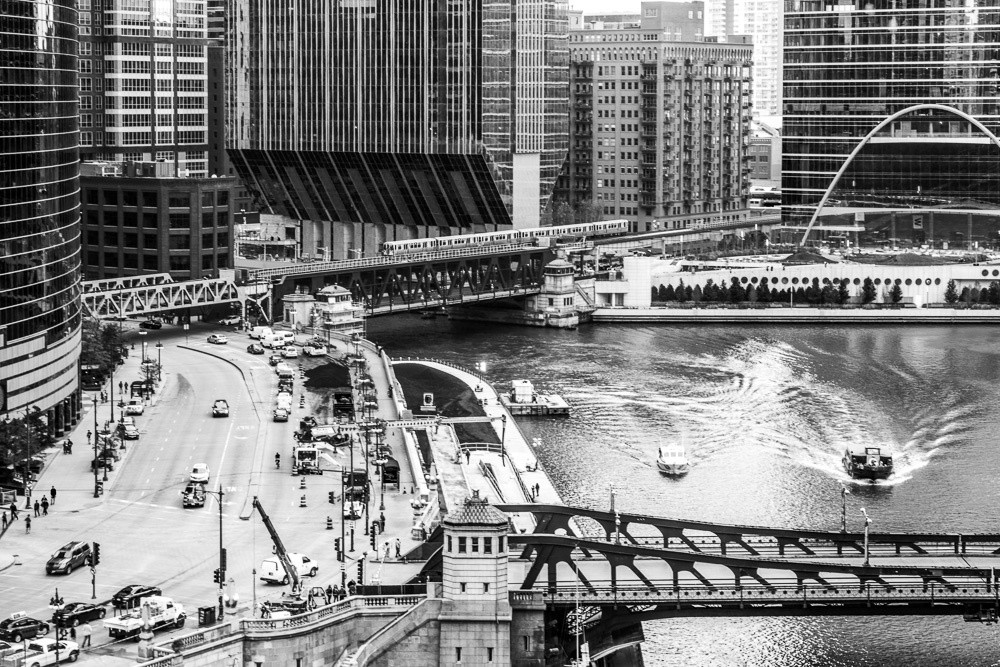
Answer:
[
  {"left": 0, "top": 381, "right": 129, "bottom": 535},
  {"left": 466, "top": 449, "right": 470, "bottom": 465},
  {"left": 70, "top": 622, "right": 77, "bottom": 642},
  {"left": 535, "top": 482, "right": 540, "bottom": 497},
  {"left": 261, "top": 512, "right": 404, "bottom": 623},
  {"left": 275, "top": 452, "right": 280, "bottom": 466},
  {"left": 81, "top": 619, "right": 93, "bottom": 648},
  {"left": 531, "top": 486, "right": 535, "bottom": 500},
  {"left": 531, "top": 395, "right": 538, "bottom": 404}
]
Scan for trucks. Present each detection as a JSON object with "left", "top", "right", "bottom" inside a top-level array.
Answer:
[{"left": 102, "top": 593, "right": 188, "bottom": 643}]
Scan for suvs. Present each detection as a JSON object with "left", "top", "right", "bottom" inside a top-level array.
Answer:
[
  {"left": 207, "top": 312, "right": 330, "bottom": 424},
  {"left": 86, "top": 395, "right": 146, "bottom": 472},
  {"left": 188, "top": 462, "right": 210, "bottom": 484},
  {"left": 44, "top": 539, "right": 91, "bottom": 577},
  {"left": 342, "top": 485, "right": 370, "bottom": 501},
  {"left": 181, "top": 481, "right": 207, "bottom": 508},
  {"left": 138, "top": 320, "right": 161, "bottom": 330}
]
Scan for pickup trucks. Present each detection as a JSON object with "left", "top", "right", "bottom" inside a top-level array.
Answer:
[{"left": 0, "top": 638, "right": 81, "bottom": 667}]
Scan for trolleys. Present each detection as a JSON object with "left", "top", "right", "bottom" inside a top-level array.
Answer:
[{"left": 64, "top": 444, "right": 72, "bottom": 454}]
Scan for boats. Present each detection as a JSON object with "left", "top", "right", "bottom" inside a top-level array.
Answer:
[
  {"left": 842, "top": 444, "right": 896, "bottom": 483},
  {"left": 420, "top": 311, "right": 436, "bottom": 320},
  {"left": 658, "top": 444, "right": 690, "bottom": 477}
]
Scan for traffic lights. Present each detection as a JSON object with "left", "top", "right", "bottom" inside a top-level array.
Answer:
[
  {"left": 86, "top": 553, "right": 92, "bottom": 567},
  {"left": 94, "top": 542, "right": 100, "bottom": 565},
  {"left": 334, "top": 539, "right": 339, "bottom": 551},
  {"left": 329, "top": 491, "right": 334, "bottom": 503},
  {"left": 213, "top": 568, "right": 220, "bottom": 584},
  {"left": 356, "top": 558, "right": 363, "bottom": 585},
  {"left": 368, "top": 529, "right": 375, "bottom": 546}
]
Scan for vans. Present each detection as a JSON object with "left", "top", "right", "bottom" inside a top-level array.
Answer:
[{"left": 259, "top": 551, "right": 319, "bottom": 585}]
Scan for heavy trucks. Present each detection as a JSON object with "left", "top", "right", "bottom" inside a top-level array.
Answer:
[{"left": 293, "top": 443, "right": 324, "bottom": 475}]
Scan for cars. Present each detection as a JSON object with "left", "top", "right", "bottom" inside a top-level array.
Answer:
[
  {"left": 111, "top": 584, "right": 162, "bottom": 609},
  {"left": 0, "top": 611, "right": 50, "bottom": 643},
  {"left": 52, "top": 602, "right": 106, "bottom": 627},
  {"left": 342, "top": 501, "right": 364, "bottom": 519},
  {"left": 0, "top": 640, "right": 25, "bottom": 658}
]
